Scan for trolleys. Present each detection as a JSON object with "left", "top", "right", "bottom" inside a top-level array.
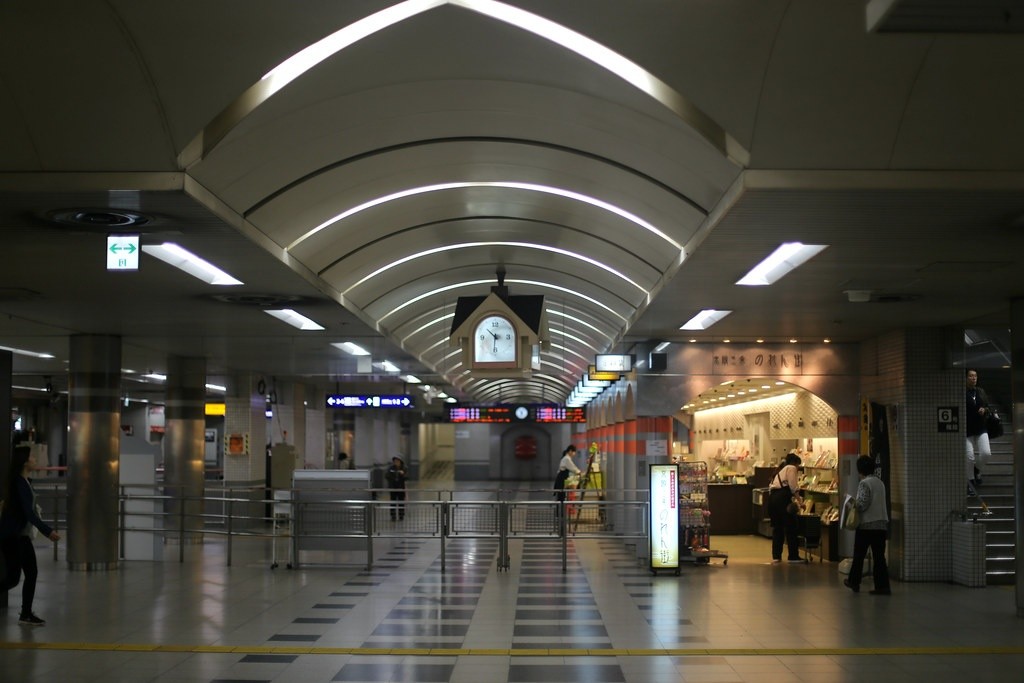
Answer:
[{"left": 796, "top": 513, "right": 825, "bottom": 565}]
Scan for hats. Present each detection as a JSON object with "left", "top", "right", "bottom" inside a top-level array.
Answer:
[{"left": 392, "top": 453, "right": 405, "bottom": 463}]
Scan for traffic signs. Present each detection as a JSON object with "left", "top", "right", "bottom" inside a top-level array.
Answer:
[{"left": 326, "top": 393, "right": 415, "bottom": 409}]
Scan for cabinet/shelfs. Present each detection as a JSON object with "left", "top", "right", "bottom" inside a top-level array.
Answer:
[{"left": 798, "top": 466, "right": 839, "bottom": 562}]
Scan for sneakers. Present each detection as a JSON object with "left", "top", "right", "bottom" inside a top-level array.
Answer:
[
  {"left": 18, "top": 614, "right": 46, "bottom": 626},
  {"left": 773, "top": 559, "right": 783, "bottom": 562},
  {"left": 788, "top": 557, "right": 807, "bottom": 563}
]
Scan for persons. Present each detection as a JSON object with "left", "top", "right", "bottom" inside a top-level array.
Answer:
[
  {"left": 769, "top": 452, "right": 807, "bottom": 563},
  {"left": 0, "top": 445, "right": 61, "bottom": 627},
  {"left": 335, "top": 452, "right": 350, "bottom": 470},
  {"left": 965, "top": 368, "right": 992, "bottom": 496},
  {"left": 843, "top": 455, "right": 892, "bottom": 597},
  {"left": 552, "top": 444, "right": 582, "bottom": 526},
  {"left": 385, "top": 453, "right": 408, "bottom": 522}
]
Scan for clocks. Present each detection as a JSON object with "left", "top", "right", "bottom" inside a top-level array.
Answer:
[{"left": 468, "top": 310, "right": 521, "bottom": 370}]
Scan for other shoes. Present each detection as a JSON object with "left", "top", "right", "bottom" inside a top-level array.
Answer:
[
  {"left": 844, "top": 578, "right": 859, "bottom": 592},
  {"left": 870, "top": 590, "right": 892, "bottom": 598},
  {"left": 391, "top": 517, "right": 397, "bottom": 521},
  {"left": 974, "top": 467, "right": 983, "bottom": 484},
  {"left": 968, "top": 479, "right": 975, "bottom": 495},
  {"left": 398, "top": 516, "right": 404, "bottom": 520}
]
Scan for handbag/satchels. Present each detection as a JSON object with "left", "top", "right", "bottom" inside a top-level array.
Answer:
[
  {"left": 845, "top": 497, "right": 860, "bottom": 530},
  {"left": 787, "top": 502, "right": 800, "bottom": 514},
  {"left": 985, "top": 405, "right": 1003, "bottom": 439}
]
